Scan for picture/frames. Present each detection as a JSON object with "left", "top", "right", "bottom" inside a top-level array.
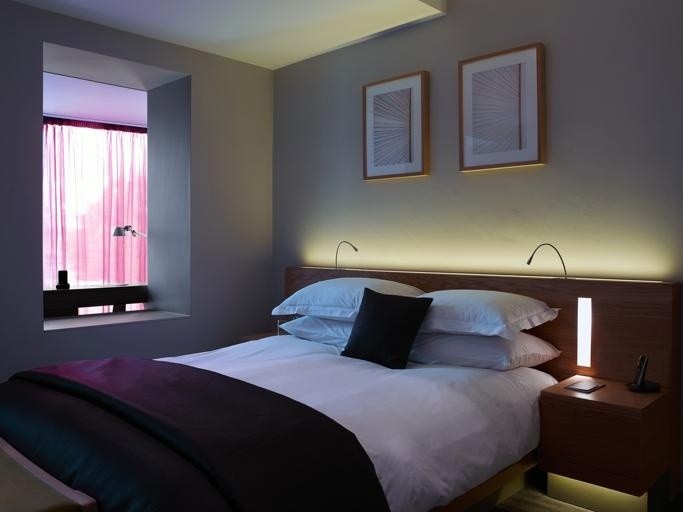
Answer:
[
  {"left": 457, "top": 40, "right": 547, "bottom": 173},
  {"left": 362, "top": 70, "right": 433, "bottom": 182}
]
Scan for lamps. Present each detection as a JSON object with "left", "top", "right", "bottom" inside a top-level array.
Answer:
[{"left": 111, "top": 224, "right": 149, "bottom": 237}]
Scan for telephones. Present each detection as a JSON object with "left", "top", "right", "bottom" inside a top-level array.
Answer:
[{"left": 628, "top": 354, "right": 660, "bottom": 393}]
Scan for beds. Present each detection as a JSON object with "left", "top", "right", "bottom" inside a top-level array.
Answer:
[{"left": 1, "top": 330, "right": 559, "bottom": 509}]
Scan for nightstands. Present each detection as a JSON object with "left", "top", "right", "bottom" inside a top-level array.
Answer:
[{"left": 541, "top": 375, "right": 671, "bottom": 497}]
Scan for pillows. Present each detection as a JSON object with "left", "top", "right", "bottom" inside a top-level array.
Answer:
[{"left": 273, "top": 277, "right": 565, "bottom": 369}]
